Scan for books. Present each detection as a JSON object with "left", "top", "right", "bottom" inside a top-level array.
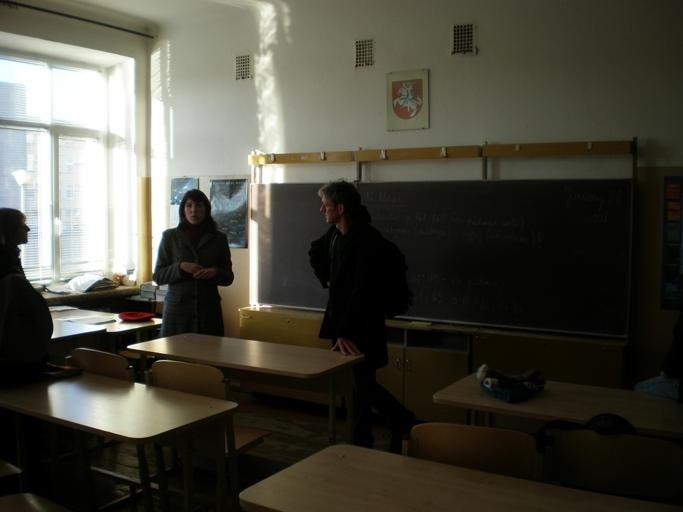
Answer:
[
  {"left": 30, "top": 273, "right": 119, "bottom": 294},
  {"left": 54, "top": 314, "right": 116, "bottom": 326},
  {"left": 139, "top": 280, "right": 168, "bottom": 302},
  {"left": 48, "top": 305, "right": 78, "bottom": 312}
]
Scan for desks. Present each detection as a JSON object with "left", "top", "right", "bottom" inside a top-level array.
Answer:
[
  {"left": 49, "top": 303, "right": 164, "bottom": 362},
  {"left": 433, "top": 374, "right": 681, "bottom": 444},
  {"left": 239, "top": 444, "right": 681, "bottom": 510},
  {"left": 123, "top": 330, "right": 363, "bottom": 440},
  {"left": 2, "top": 368, "right": 245, "bottom": 510}
]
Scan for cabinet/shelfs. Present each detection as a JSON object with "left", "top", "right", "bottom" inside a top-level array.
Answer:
[
  {"left": 377, "top": 325, "right": 472, "bottom": 426},
  {"left": 472, "top": 333, "right": 617, "bottom": 384},
  {"left": 241, "top": 315, "right": 344, "bottom": 399}
]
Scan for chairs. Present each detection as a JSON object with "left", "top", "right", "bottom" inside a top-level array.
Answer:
[
  {"left": 550, "top": 431, "right": 682, "bottom": 500},
  {"left": 408, "top": 421, "right": 544, "bottom": 479},
  {"left": 70, "top": 350, "right": 166, "bottom": 491},
  {"left": 152, "top": 357, "right": 273, "bottom": 506}
]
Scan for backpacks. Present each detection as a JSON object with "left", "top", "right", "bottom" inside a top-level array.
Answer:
[{"left": 377, "top": 231, "right": 412, "bottom": 317}]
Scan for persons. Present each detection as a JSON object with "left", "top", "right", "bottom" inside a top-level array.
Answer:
[
  {"left": 153, "top": 189, "right": 235, "bottom": 337},
  {"left": 308, "top": 181, "right": 425, "bottom": 448},
  {"left": 0, "top": 207, "right": 79, "bottom": 386}
]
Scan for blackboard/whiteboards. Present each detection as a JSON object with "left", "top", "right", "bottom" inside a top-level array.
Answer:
[{"left": 248, "top": 177, "right": 636, "bottom": 338}]
